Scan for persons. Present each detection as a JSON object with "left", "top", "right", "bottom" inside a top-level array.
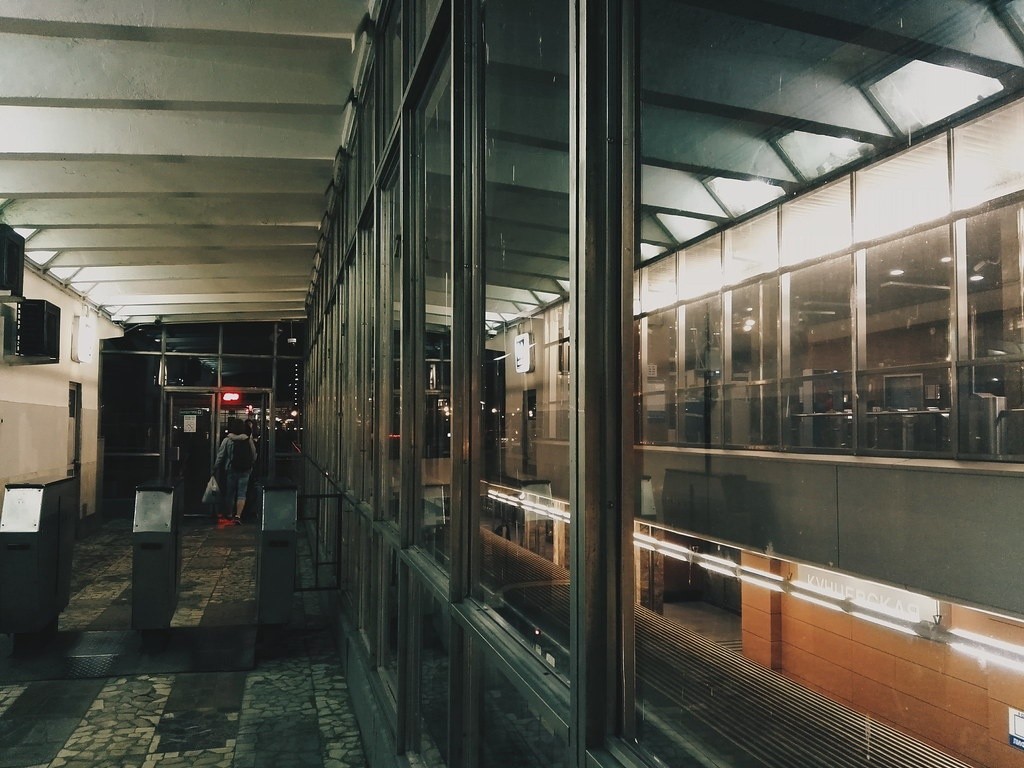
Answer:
[
  {"left": 495, "top": 519, "right": 511, "bottom": 541},
  {"left": 245, "top": 419, "right": 253, "bottom": 428},
  {"left": 274, "top": 421, "right": 288, "bottom": 449},
  {"left": 210, "top": 419, "right": 253, "bottom": 526}
]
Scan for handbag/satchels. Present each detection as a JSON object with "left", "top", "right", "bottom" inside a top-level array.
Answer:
[{"left": 201, "top": 476, "right": 222, "bottom": 504}]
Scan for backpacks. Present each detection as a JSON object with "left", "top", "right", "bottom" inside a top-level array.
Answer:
[{"left": 227, "top": 436, "right": 252, "bottom": 473}]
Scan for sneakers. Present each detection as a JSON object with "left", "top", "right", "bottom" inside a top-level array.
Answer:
[
  {"left": 234, "top": 516, "right": 242, "bottom": 525},
  {"left": 225, "top": 515, "right": 232, "bottom": 520}
]
